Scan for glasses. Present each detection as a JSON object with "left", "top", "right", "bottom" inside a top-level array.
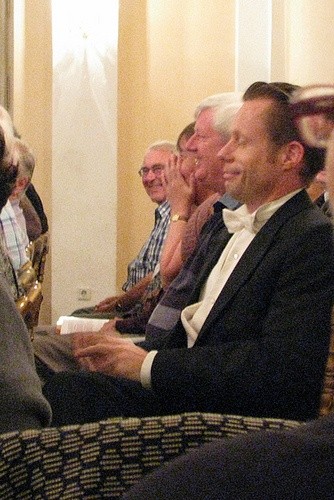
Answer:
[
  {"left": 139, "top": 165, "right": 164, "bottom": 177},
  {"left": 287, "top": 84, "right": 334, "bottom": 148}
]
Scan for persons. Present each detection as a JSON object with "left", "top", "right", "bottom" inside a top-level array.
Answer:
[{"left": 0, "top": 81, "right": 334, "bottom": 500}]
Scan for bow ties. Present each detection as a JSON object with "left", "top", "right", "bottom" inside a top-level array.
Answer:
[{"left": 222, "top": 205, "right": 264, "bottom": 234}]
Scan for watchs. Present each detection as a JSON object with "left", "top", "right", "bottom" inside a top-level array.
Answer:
[{"left": 169, "top": 214, "right": 188, "bottom": 224}]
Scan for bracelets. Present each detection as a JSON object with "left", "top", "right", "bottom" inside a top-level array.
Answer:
[{"left": 114, "top": 300, "right": 125, "bottom": 311}]
